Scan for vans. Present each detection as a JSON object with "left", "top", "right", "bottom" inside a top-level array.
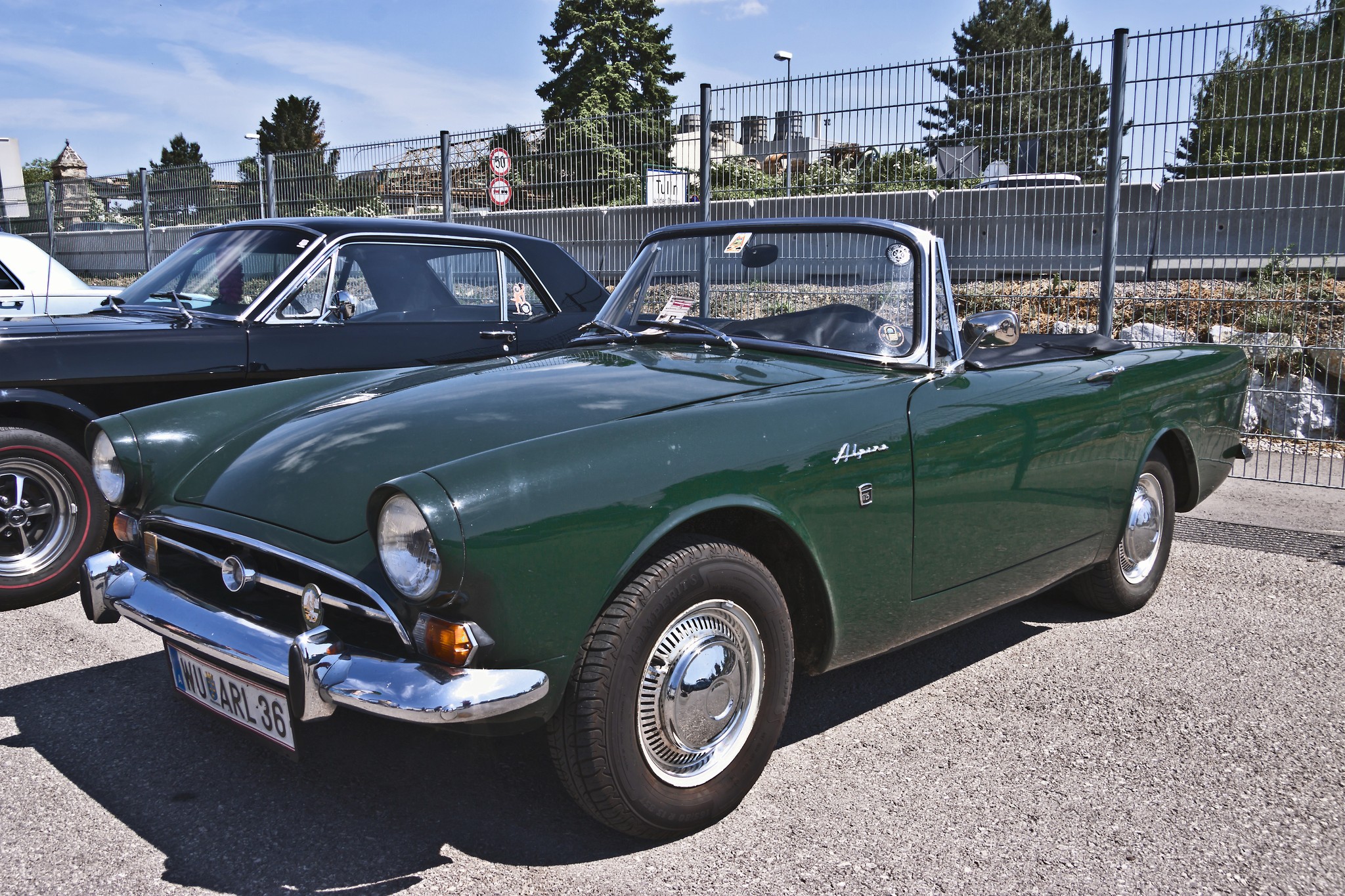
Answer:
[
  {"left": 0, "top": 232, "right": 216, "bottom": 317},
  {"left": 62, "top": 222, "right": 141, "bottom": 233}
]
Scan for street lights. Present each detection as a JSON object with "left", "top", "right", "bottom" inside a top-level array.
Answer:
[
  {"left": 244, "top": 133, "right": 264, "bottom": 219},
  {"left": 354, "top": 143, "right": 395, "bottom": 196},
  {"left": 775, "top": 51, "right": 793, "bottom": 196}
]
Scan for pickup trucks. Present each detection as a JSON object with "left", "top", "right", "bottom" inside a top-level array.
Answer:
[{"left": 81, "top": 218, "right": 1252, "bottom": 847}]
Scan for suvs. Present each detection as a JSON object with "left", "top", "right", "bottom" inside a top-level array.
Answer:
[
  {"left": 974, "top": 172, "right": 1082, "bottom": 189},
  {"left": 0, "top": 217, "right": 741, "bottom": 612}
]
